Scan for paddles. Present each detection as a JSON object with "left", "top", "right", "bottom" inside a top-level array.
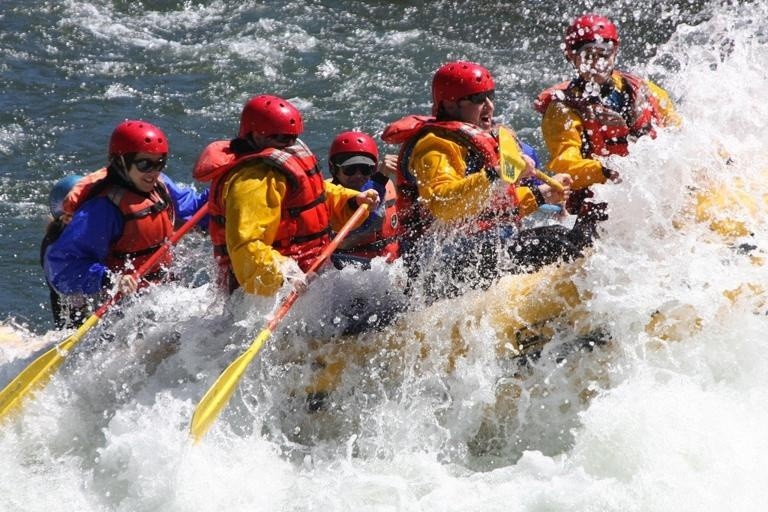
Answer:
[
  {"left": 191, "top": 191, "right": 373, "bottom": 442},
  {"left": 0, "top": 198, "right": 209, "bottom": 422},
  {"left": 497, "top": 126, "right": 565, "bottom": 193}
]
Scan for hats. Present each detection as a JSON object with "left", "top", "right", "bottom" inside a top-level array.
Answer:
[
  {"left": 334, "top": 152, "right": 375, "bottom": 168},
  {"left": 572, "top": 40, "right": 614, "bottom": 53}
]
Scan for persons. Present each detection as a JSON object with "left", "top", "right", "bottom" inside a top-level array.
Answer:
[
  {"left": 381, "top": 60, "right": 577, "bottom": 303},
  {"left": 319, "top": 131, "right": 398, "bottom": 274},
  {"left": 189, "top": 93, "right": 379, "bottom": 298},
  {"left": 39, "top": 121, "right": 208, "bottom": 325},
  {"left": 531, "top": 12, "right": 732, "bottom": 235}
]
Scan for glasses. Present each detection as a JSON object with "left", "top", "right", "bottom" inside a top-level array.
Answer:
[
  {"left": 263, "top": 133, "right": 298, "bottom": 143},
  {"left": 341, "top": 164, "right": 373, "bottom": 176},
  {"left": 459, "top": 89, "right": 495, "bottom": 104},
  {"left": 130, "top": 156, "right": 166, "bottom": 173}
]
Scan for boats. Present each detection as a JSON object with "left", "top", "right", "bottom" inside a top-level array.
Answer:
[{"left": 1, "top": 149, "right": 766, "bottom": 459}]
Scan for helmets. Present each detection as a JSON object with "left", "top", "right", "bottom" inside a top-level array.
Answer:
[
  {"left": 565, "top": 14, "right": 618, "bottom": 50},
  {"left": 48, "top": 175, "right": 83, "bottom": 219},
  {"left": 329, "top": 131, "right": 378, "bottom": 172},
  {"left": 109, "top": 120, "right": 168, "bottom": 157},
  {"left": 239, "top": 94, "right": 303, "bottom": 139},
  {"left": 431, "top": 62, "right": 494, "bottom": 117}
]
